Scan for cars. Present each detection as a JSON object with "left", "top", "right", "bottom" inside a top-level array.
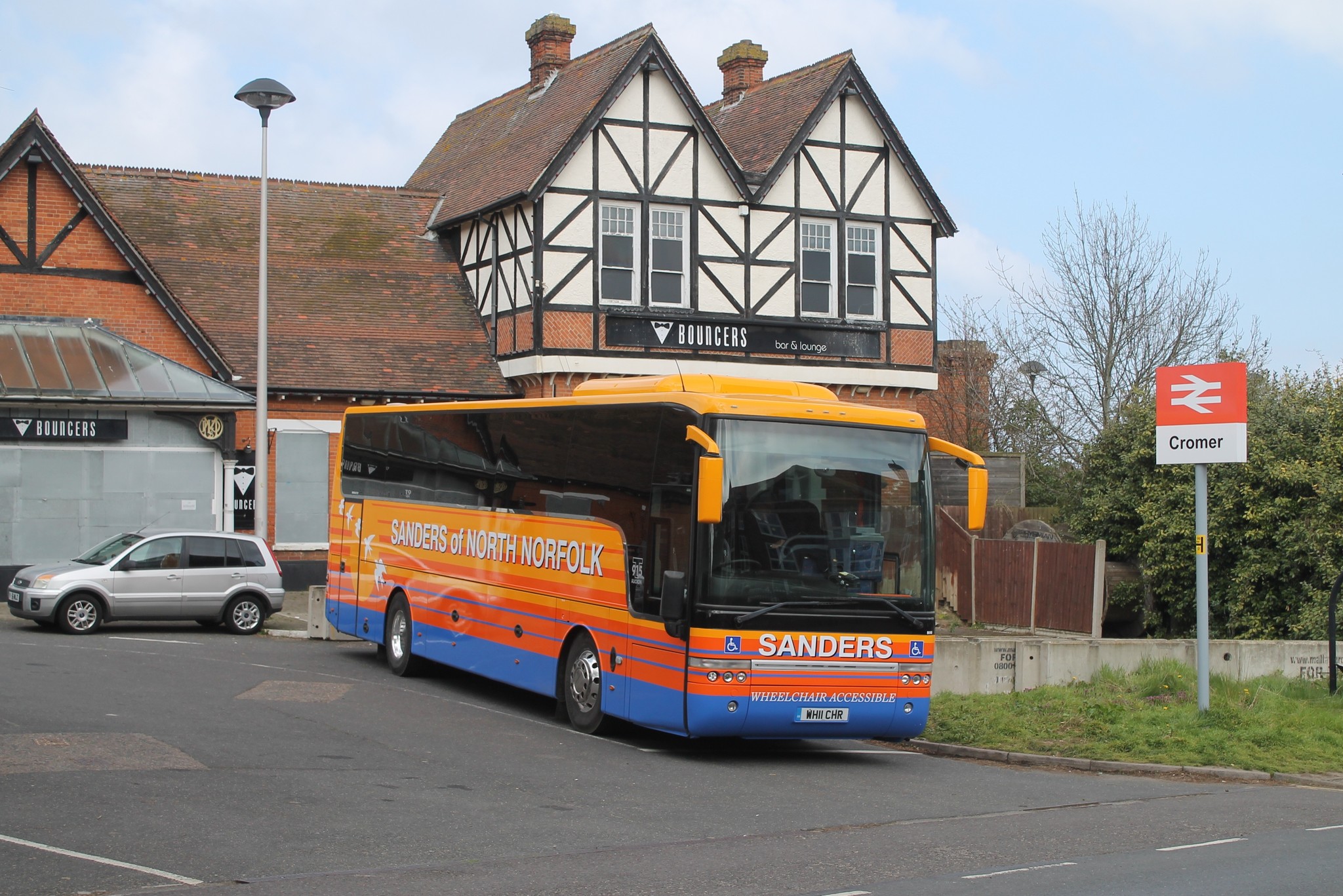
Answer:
[{"left": 7, "top": 525, "right": 285, "bottom": 636}]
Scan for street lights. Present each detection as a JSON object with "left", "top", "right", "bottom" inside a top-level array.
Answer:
[{"left": 233, "top": 77, "right": 297, "bottom": 540}]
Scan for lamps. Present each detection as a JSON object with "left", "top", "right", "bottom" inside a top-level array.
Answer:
[{"left": 241, "top": 437, "right": 251, "bottom": 453}]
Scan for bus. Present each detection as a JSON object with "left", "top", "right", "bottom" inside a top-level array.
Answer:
[{"left": 323, "top": 372, "right": 989, "bottom": 743}]
